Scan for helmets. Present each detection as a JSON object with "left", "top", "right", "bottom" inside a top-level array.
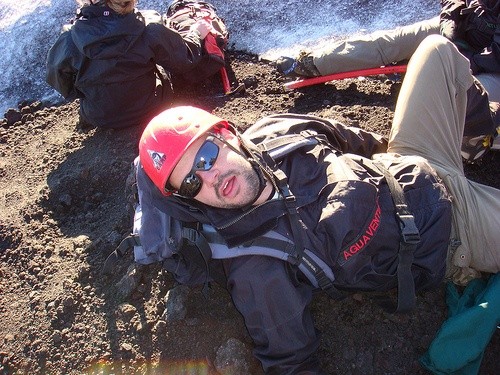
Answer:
[{"left": 138, "top": 105, "right": 229, "bottom": 196}]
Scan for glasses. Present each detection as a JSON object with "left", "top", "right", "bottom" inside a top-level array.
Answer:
[{"left": 178, "top": 134, "right": 220, "bottom": 198}]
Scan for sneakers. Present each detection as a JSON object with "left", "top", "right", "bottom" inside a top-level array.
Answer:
[{"left": 461, "top": 101, "right": 500, "bottom": 166}]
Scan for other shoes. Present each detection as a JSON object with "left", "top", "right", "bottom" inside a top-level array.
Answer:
[{"left": 293, "top": 52, "right": 321, "bottom": 78}]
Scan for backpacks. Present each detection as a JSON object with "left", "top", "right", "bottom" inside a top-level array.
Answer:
[
  {"left": 440, "top": 0, "right": 496, "bottom": 51},
  {"left": 130, "top": 130, "right": 345, "bottom": 300},
  {"left": 165, "top": 0, "right": 229, "bottom": 89}
]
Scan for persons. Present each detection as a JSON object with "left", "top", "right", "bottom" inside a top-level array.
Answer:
[
  {"left": 40, "top": 1, "right": 214, "bottom": 129},
  {"left": 139, "top": 34, "right": 500, "bottom": 375},
  {"left": 274, "top": 0, "right": 500, "bottom": 105}
]
